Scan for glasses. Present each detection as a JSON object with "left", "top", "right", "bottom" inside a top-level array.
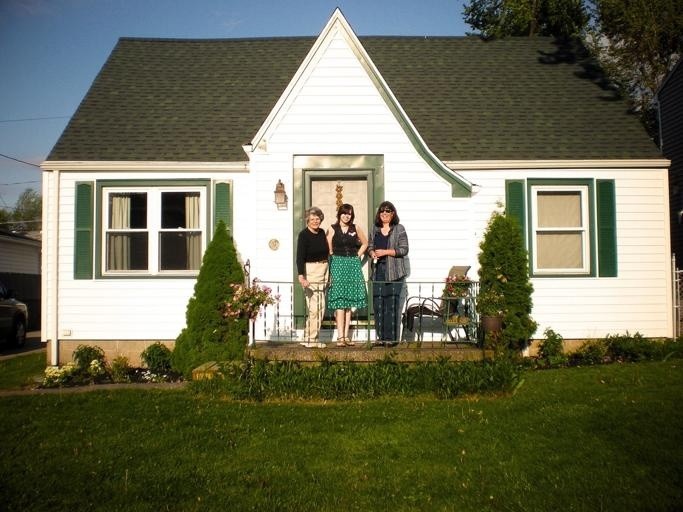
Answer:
[{"left": 379, "top": 209, "right": 392, "bottom": 213}]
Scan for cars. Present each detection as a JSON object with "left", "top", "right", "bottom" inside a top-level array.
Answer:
[{"left": 0, "top": 287, "right": 29, "bottom": 349}]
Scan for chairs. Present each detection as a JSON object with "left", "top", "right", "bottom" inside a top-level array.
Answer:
[{"left": 402, "top": 265, "right": 473, "bottom": 348}]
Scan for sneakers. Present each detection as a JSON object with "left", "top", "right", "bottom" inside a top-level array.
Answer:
[
  {"left": 375, "top": 338, "right": 397, "bottom": 346},
  {"left": 304, "top": 340, "right": 326, "bottom": 348}
]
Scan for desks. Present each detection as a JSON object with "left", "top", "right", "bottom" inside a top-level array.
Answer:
[{"left": 443, "top": 319, "right": 479, "bottom": 348}]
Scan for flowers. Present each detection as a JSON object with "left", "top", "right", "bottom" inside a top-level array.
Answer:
[
  {"left": 222, "top": 282, "right": 282, "bottom": 320},
  {"left": 443, "top": 272, "right": 473, "bottom": 300}
]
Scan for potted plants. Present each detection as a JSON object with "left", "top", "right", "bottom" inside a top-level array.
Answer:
[{"left": 475, "top": 289, "right": 507, "bottom": 336}]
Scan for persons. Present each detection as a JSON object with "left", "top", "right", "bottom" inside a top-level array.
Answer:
[
  {"left": 297, "top": 207, "right": 329, "bottom": 348},
  {"left": 367, "top": 201, "right": 409, "bottom": 346},
  {"left": 325, "top": 204, "right": 368, "bottom": 346}
]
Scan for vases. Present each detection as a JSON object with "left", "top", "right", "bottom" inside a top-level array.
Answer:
[{"left": 456, "top": 297, "right": 465, "bottom": 319}]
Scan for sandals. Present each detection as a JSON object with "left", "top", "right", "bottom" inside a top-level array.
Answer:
[
  {"left": 345, "top": 337, "right": 355, "bottom": 346},
  {"left": 336, "top": 337, "right": 346, "bottom": 347}
]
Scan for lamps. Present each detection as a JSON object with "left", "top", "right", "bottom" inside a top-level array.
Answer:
[{"left": 272, "top": 179, "right": 288, "bottom": 205}]
